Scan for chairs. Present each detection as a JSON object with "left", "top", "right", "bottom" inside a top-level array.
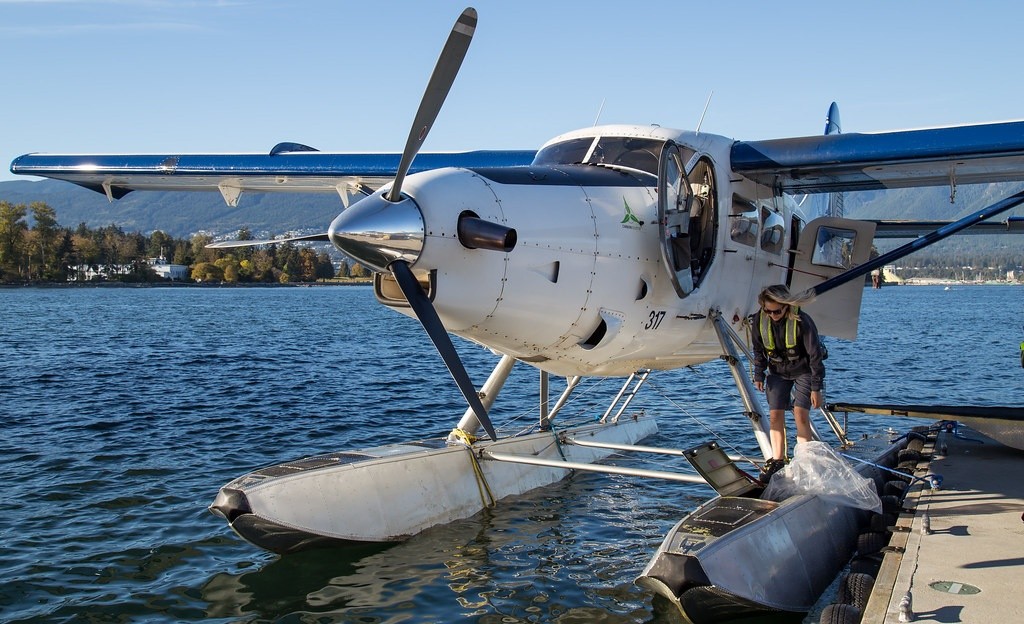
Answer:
[{"left": 680, "top": 195, "right": 710, "bottom": 250}]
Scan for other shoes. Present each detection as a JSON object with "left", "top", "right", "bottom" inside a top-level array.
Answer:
[{"left": 759, "top": 458, "right": 784, "bottom": 482}]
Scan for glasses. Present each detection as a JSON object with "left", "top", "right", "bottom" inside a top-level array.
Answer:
[{"left": 763, "top": 305, "right": 786, "bottom": 315}]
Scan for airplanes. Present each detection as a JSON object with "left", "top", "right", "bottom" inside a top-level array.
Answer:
[{"left": 7, "top": 6, "right": 1023, "bottom": 624}]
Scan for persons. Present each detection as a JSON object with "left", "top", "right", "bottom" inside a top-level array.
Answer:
[{"left": 751, "top": 284, "right": 826, "bottom": 482}]
